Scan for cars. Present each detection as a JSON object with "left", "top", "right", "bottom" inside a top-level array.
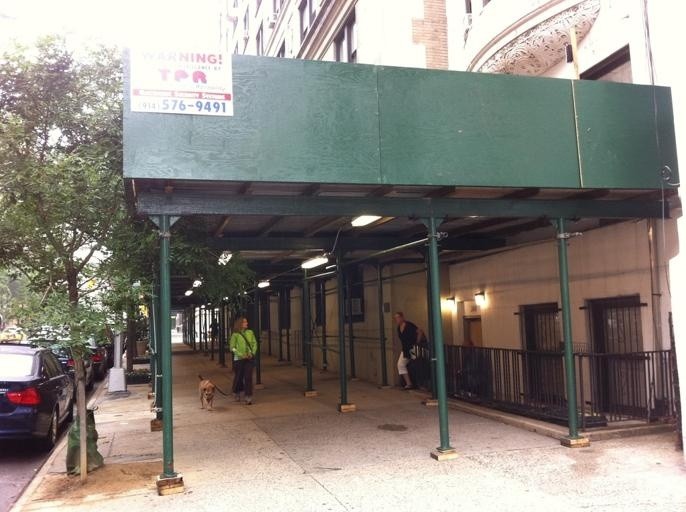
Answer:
[{"left": 0, "top": 324, "right": 127, "bottom": 452}]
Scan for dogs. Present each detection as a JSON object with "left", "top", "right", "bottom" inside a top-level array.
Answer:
[{"left": 198, "top": 375, "right": 216, "bottom": 409}]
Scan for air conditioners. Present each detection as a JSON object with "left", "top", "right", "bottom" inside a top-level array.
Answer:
[{"left": 266, "top": 13, "right": 279, "bottom": 28}]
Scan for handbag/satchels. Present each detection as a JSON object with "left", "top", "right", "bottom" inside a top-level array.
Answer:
[
  {"left": 406, "top": 358, "right": 430, "bottom": 384},
  {"left": 249, "top": 355, "right": 257, "bottom": 368}
]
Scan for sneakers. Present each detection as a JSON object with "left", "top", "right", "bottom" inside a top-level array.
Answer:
[
  {"left": 404, "top": 384, "right": 414, "bottom": 389},
  {"left": 232, "top": 392, "right": 254, "bottom": 405}
]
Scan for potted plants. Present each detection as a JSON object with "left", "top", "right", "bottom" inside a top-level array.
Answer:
[{"left": 135, "top": 316, "right": 150, "bottom": 357}]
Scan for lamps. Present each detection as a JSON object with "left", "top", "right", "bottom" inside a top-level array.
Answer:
[
  {"left": 446, "top": 297, "right": 455, "bottom": 310},
  {"left": 474, "top": 292, "right": 485, "bottom": 306}
]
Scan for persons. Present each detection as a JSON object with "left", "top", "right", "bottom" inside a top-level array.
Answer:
[
  {"left": 228, "top": 316, "right": 258, "bottom": 404},
  {"left": 393, "top": 311, "right": 429, "bottom": 390},
  {"left": 209, "top": 319, "right": 220, "bottom": 338}
]
